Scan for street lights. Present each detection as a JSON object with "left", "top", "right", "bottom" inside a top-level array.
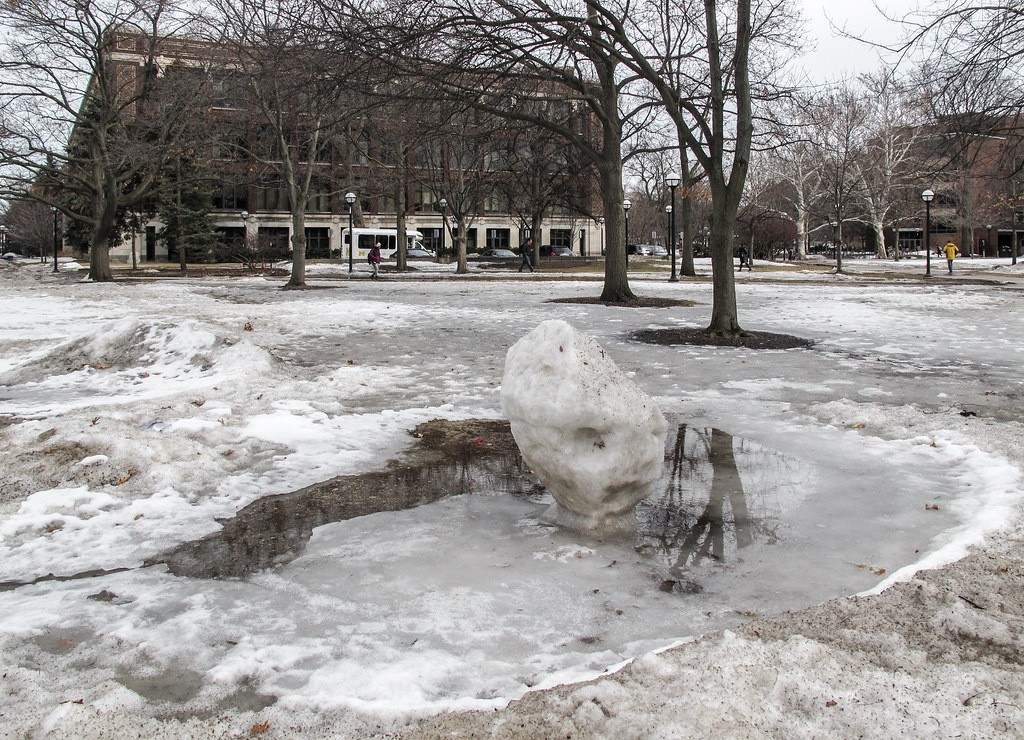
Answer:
[
  {"left": 0, "top": 224, "right": 9, "bottom": 256},
  {"left": 780, "top": 211, "right": 788, "bottom": 262},
  {"left": 439, "top": 198, "right": 449, "bottom": 255},
  {"left": 345, "top": 192, "right": 357, "bottom": 272},
  {"left": 663, "top": 172, "right": 680, "bottom": 282},
  {"left": 665, "top": 204, "right": 673, "bottom": 255},
  {"left": 623, "top": 198, "right": 633, "bottom": 269},
  {"left": 240, "top": 210, "right": 249, "bottom": 250},
  {"left": 49, "top": 204, "right": 61, "bottom": 272},
  {"left": 921, "top": 190, "right": 935, "bottom": 279},
  {"left": 832, "top": 221, "right": 838, "bottom": 259},
  {"left": 986, "top": 224, "right": 993, "bottom": 256}
]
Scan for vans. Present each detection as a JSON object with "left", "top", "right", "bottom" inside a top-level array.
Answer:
[{"left": 627, "top": 243, "right": 668, "bottom": 258}]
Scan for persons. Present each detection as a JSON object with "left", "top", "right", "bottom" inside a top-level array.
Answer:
[
  {"left": 369, "top": 243, "right": 381, "bottom": 280},
  {"left": 944, "top": 241, "right": 959, "bottom": 274},
  {"left": 736, "top": 243, "right": 752, "bottom": 272},
  {"left": 937, "top": 245, "right": 943, "bottom": 258},
  {"left": 517, "top": 238, "right": 538, "bottom": 273}
]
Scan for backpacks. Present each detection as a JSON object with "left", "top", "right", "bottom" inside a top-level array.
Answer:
[
  {"left": 939, "top": 247, "right": 943, "bottom": 250},
  {"left": 367, "top": 249, "right": 375, "bottom": 265}
]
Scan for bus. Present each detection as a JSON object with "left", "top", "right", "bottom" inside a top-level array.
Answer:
[{"left": 341, "top": 227, "right": 435, "bottom": 259}]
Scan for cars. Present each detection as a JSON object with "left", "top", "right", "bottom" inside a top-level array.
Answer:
[
  {"left": 390, "top": 249, "right": 431, "bottom": 258},
  {"left": 477, "top": 248, "right": 517, "bottom": 261},
  {"left": 757, "top": 245, "right": 789, "bottom": 259},
  {"left": 539, "top": 245, "right": 575, "bottom": 258}
]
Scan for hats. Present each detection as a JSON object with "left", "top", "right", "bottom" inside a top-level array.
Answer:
[{"left": 374, "top": 242, "right": 381, "bottom": 247}]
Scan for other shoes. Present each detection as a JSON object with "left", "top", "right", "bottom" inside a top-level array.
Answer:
[
  {"left": 737, "top": 270, "right": 742, "bottom": 271},
  {"left": 748, "top": 268, "right": 751, "bottom": 270}
]
